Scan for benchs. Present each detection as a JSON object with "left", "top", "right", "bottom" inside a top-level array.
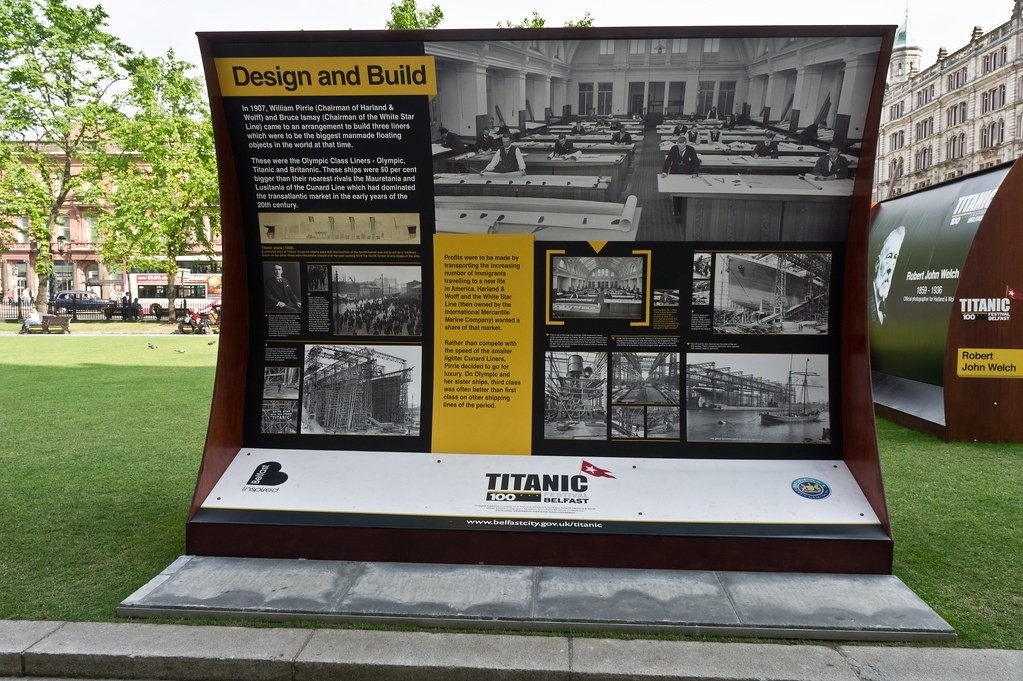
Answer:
[
  {"left": 177, "top": 316, "right": 221, "bottom": 334},
  {"left": 19, "top": 315, "right": 73, "bottom": 335},
  {"left": 153, "top": 308, "right": 193, "bottom": 321},
  {"left": 101, "top": 307, "right": 144, "bottom": 322}
]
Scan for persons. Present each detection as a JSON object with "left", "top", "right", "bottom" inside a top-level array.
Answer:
[
  {"left": 439, "top": 128, "right": 467, "bottom": 172},
  {"left": 308, "top": 266, "right": 327, "bottom": 290},
  {"left": 481, "top": 134, "right": 526, "bottom": 174},
  {"left": 7, "top": 287, "right": 42, "bottom": 334},
  {"left": 570, "top": 120, "right": 585, "bottom": 136},
  {"left": 751, "top": 133, "right": 778, "bottom": 159},
  {"left": 266, "top": 264, "right": 301, "bottom": 312},
  {"left": 662, "top": 137, "right": 700, "bottom": 216},
  {"left": 488, "top": 117, "right": 509, "bottom": 145},
  {"left": 660, "top": 291, "right": 674, "bottom": 304},
  {"left": 475, "top": 129, "right": 495, "bottom": 154},
  {"left": 869, "top": 225, "right": 905, "bottom": 331},
  {"left": 334, "top": 296, "right": 422, "bottom": 336},
  {"left": 812, "top": 144, "right": 848, "bottom": 180},
  {"left": 587, "top": 107, "right": 743, "bottom": 145},
  {"left": 554, "top": 134, "right": 573, "bottom": 158},
  {"left": 797, "top": 121, "right": 827, "bottom": 144},
  {"left": 178, "top": 308, "right": 220, "bottom": 334},
  {"left": 560, "top": 286, "right": 641, "bottom": 310},
  {"left": 122, "top": 291, "right": 143, "bottom": 322}
]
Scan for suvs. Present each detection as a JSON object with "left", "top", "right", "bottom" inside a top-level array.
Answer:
[{"left": 54, "top": 291, "right": 117, "bottom": 313}]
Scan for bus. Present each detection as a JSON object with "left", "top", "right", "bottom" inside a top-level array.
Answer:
[{"left": 128, "top": 273, "right": 222, "bottom": 316}]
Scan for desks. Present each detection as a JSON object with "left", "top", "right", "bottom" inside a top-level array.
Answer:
[
  {"left": 655, "top": 116, "right": 860, "bottom": 242},
  {"left": 431, "top": 119, "right": 643, "bottom": 241}
]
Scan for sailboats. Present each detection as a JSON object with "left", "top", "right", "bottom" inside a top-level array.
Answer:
[{"left": 759, "top": 354, "right": 824, "bottom": 425}]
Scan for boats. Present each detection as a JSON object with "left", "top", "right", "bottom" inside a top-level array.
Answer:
[{"left": 727, "top": 254, "right": 825, "bottom": 321}]
